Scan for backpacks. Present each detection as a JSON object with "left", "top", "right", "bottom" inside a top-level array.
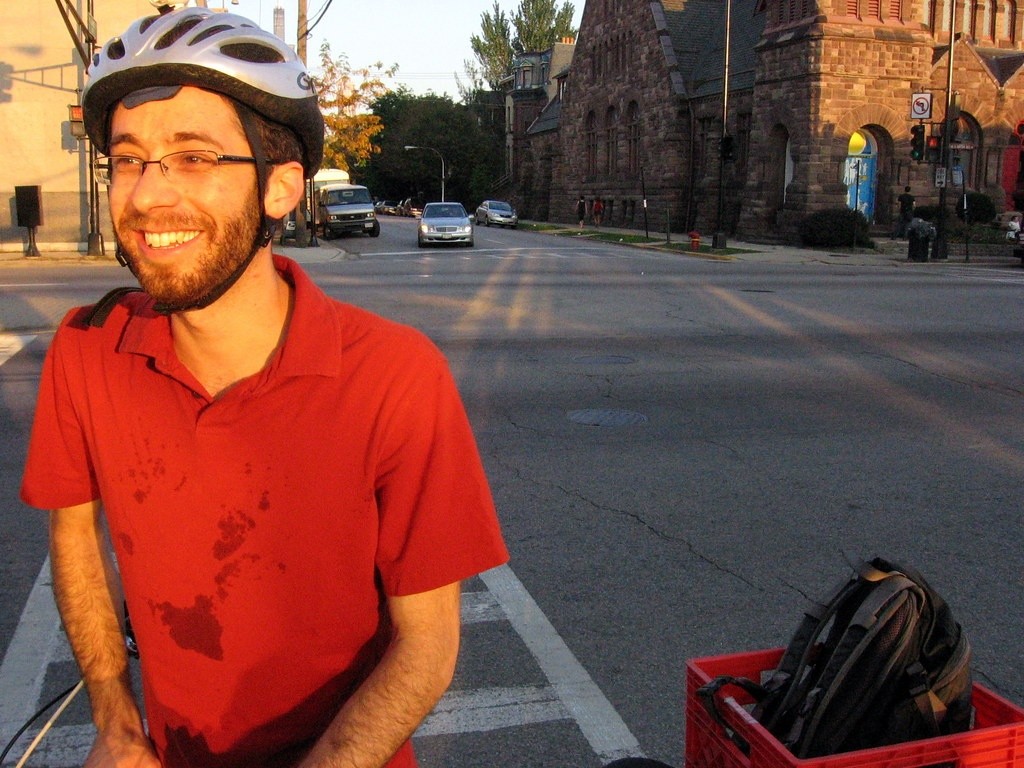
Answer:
[{"left": 700, "top": 553, "right": 976, "bottom": 768}]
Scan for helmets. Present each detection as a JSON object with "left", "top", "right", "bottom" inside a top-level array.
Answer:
[{"left": 81, "top": 6, "right": 324, "bottom": 180}]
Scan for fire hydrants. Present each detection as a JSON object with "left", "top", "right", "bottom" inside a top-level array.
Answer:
[{"left": 687, "top": 230, "right": 700, "bottom": 250}]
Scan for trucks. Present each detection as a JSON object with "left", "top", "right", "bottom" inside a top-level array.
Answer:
[{"left": 316, "top": 183, "right": 381, "bottom": 241}]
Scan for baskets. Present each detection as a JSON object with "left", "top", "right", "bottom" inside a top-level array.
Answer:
[{"left": 683, "top": 641, "right": 1024, "bottom": 767}]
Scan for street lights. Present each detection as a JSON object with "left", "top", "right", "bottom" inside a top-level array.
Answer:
[{"left": 404, "top": 145, "right": 445, "bottom": 203}]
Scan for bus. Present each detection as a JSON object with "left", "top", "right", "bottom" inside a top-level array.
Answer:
[{"left": 306, "top": 169, "right": 350, "bottom": 225}]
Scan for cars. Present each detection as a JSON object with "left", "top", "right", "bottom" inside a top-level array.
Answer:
[
  {"left": 415, "top": 202, "right": 475, "bottom": 247},
  {"left": 476, "top": 200, "right": 519, "bottom": 229},
  {"left": 375, "top": 197, "right": 426, "bottom": 218}
]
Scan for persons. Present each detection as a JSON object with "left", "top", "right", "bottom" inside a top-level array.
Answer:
[
  {"left": 575, "top": 196, "right": 588, "bottom": 229},
  {"left": 1005, "top": 216, "right": 1021, "bottom": 248},
  {"left": 592, "top": 197, "right": 603, "bottom": 228},
  {"left": 893, "top": 186, "right": 916, "bottom": 242},
  {"left": 19, "top": 7, "right": 509, "bottom": 768}
]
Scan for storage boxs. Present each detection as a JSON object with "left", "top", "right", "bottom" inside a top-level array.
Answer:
[{"left": 682, "top": 647, "right": 1024, "bottom": 768}]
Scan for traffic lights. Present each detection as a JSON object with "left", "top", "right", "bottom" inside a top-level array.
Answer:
[
  {"left": 68, "top": 105, "right": 84, "bottom": 123},
  {"left": 926, "top": 135, "right": 941, "bottom": 150},
  {"left": 909, "top": 124, "right": 925, "bottom": 161}
]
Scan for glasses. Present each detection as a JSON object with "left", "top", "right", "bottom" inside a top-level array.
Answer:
[{"left": 90, "top": 149, "right": 279, "bottom": 186}]
